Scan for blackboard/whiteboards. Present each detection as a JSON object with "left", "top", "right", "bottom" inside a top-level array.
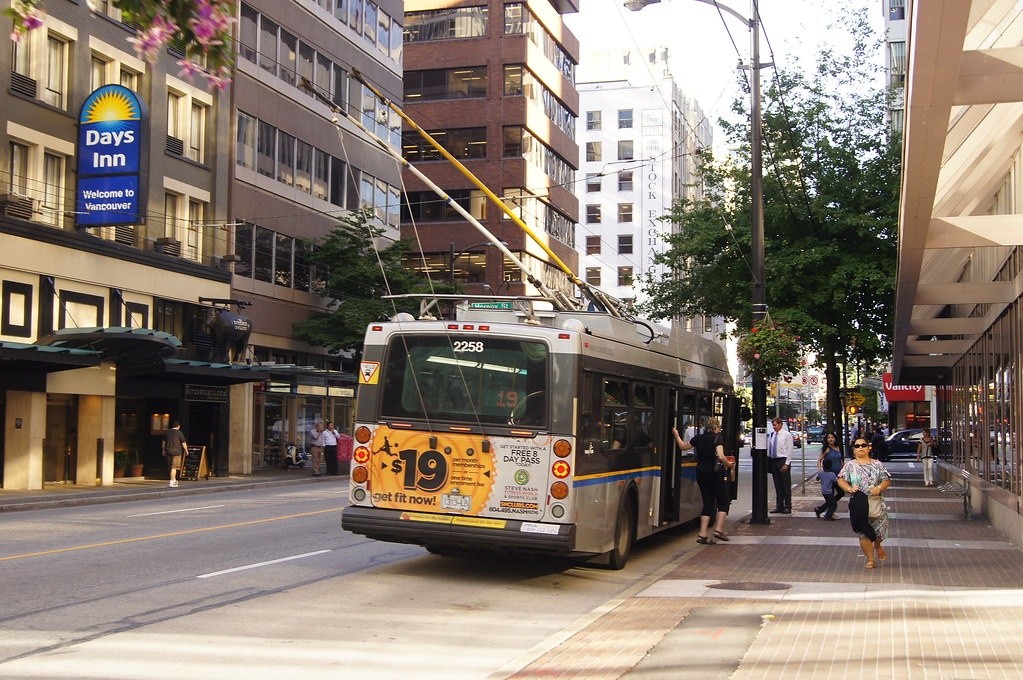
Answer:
[{"left": 179, "top": 446, "right": 209, "bottom": 478}]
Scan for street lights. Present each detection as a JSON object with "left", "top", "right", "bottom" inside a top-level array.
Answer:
[
  {"left": 623, "top": 0, "right": 771, "bottom": 524},
  {"left": 449, "top": 242, "right": 509, "bottom": 321}
]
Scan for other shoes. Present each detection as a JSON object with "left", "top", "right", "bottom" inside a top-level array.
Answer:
[
  {"left": 314, "top": 474, "right": 321, "bottom": 477},
  {"left": 824, "top": 517, "right": 835, "bottom": 521},
  {"left": 815, "top": 507, "right": 821, "bottom": 518},
  {"left": 770, "top": 509, "right": 782, "bottom": 513},
  {"left": 930, "top": 481, "right": 933, "bottom": 485},
  {"left": 782, "top": 509, "right": 790, "bottom": 514},
  {"left": 833, "top": 513, "right": 841, "bottom": 520},
  {"left": 925, "top": 483, "right": 929, "bottom": 486},
  {"left": 169, "top": 481, "right": 178, "bottom": 488}
]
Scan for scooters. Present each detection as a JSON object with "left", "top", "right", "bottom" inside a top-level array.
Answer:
[{"left": 283, "top": 437, "right": 308, "bottom": 469}]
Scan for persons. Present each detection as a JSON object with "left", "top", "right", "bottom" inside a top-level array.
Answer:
[
  {"left": 917, "top": 429, "right": 935, "bottom": 487},
  {"left": 813, "top": 432, "right": 845, "bottom": 519},
  {"left": 848, "top": 422, "right": 898, "bottom": 461},
  {"left": 816, "top": 459, "right": 838, "bottom": 521},
  {"left": 162, "top": 421, "right": 189, "bottom": 488},
  {"left": 766, "top": 418, "right": 793, "bottom": 514},
  {"left": 321, "top": 422, "right": 340, "bottom": 475},
  {"left": 308, "top": 423, "right": 323, "bottom": 476},
  {"left": 838, "top": 437, "right": 891, "bottom": 569},
  {"left": 682, "top": 418, "right": 706, "bottom": 456},
  {"left": 672, "top": 417, "right": 736, "bottom": 544}
]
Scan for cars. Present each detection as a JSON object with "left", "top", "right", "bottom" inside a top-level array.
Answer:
[
  {"left": 807, "top": 425, "right": 824, "bottom": 444},
  {"left": 883, "top": 428, "right": 938, "bottom": 461},
  {"left": 842, "top": 424, "right": 852, "bottom": 445},
  {"left": 740, "top": 421, "right": 801, "bottom": 448}
]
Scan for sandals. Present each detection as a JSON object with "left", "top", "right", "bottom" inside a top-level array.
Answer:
[
  {"left": 696, "top": 535, "right": 716, "bottom": 544},
  {"left": 714, "top": 530, "right": 729, "bottom": 541},
  {"left": 874, "top": 543, "right": 887, "bottom": 561},
  {"left": 865, "top": 560, "right": 876, "bottom": 568}
]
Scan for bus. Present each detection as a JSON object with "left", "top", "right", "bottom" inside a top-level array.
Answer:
[{"left": 300, "top": 64, "right": 751, "bottom": 570}]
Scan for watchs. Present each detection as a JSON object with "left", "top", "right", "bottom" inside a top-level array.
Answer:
[{"left": 675, "top": 435, "right": 679, "bottom": 439}]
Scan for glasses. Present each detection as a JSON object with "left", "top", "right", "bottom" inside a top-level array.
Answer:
[{"left": 854, "top": 444, "right": 869, "bottom": 448}]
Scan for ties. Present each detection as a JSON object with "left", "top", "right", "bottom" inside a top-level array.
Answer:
[{"left": 772, "top": 433, "right": 778, "bottom": 459}]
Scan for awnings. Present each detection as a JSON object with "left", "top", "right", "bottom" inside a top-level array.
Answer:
[{"left": 0, "top": 275, "right": 270, "bottom": 385}]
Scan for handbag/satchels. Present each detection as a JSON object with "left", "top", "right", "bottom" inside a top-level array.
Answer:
[
  {"left": 868, "top": 495, "right": 885, "bottom": 519},
  {"left": 714, "top": 435, "right": 724, "bottom": 473},
  {"left": 767, "top": 456, "right": 773, "bottom": 474}
]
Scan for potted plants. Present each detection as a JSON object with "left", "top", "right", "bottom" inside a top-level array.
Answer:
[
  {"left": 113, "top": 451, "right": 129, "bottom": 477},
  {"left": 129, "top": 430, "right": 145, "bottom": 477}
]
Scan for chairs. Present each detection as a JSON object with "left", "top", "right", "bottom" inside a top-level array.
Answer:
[{"left": 938, "top": 470, "right": 973, "bottom": 520}]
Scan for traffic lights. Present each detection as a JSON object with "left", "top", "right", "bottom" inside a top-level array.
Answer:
[{"left": 850, "top": 406, "right": 859, "bottom": 414}]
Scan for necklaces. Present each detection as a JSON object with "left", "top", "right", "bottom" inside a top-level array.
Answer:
[{"left": 855, "top": 458, "right": 871, "bottom": 477}]
